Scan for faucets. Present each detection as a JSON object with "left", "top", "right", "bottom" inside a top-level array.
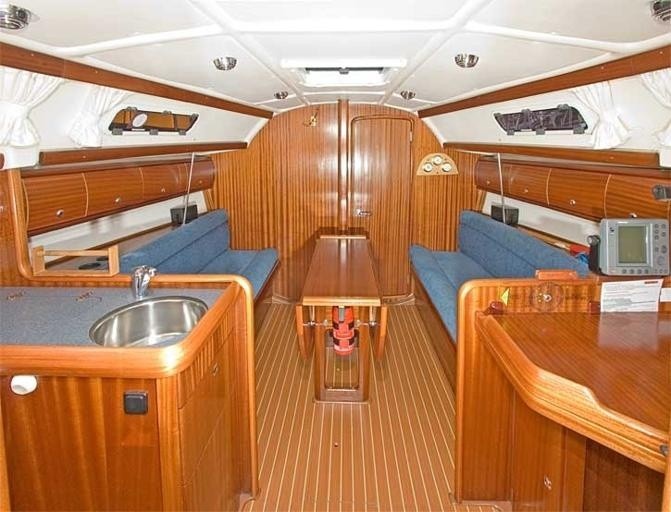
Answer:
[{"left": 130, "top": 262, "right": 157, "bottom": 300}]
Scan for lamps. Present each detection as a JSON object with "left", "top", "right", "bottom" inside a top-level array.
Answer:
[{"left": 417, "top": 153, "right": 459, "bottom": 176}]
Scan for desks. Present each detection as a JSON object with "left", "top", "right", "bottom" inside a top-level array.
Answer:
[{"left": 295, "top": 234, "right": 388, "bottom": 405}]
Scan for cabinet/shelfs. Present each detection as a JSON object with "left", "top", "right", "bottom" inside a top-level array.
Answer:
[
  {"left": 474, "top": 156, "right": 671, "bottom": 234},
  {"left": 20, "top": 155, "right": 215, "bottom": 238},
  {"left": 455, "top": 276, "right": 671, "bottom": 512}
]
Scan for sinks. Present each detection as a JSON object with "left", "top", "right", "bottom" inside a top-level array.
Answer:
[{"left": 87, "top": 293, "right": 209, "bottom": 350}]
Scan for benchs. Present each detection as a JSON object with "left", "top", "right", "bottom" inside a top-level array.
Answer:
[
  {"left": 96, "top": 208, "right": 280, "bottom": 305},
  {"left": 408, "top": 210, "right": 589, "bottom": 394}
]
